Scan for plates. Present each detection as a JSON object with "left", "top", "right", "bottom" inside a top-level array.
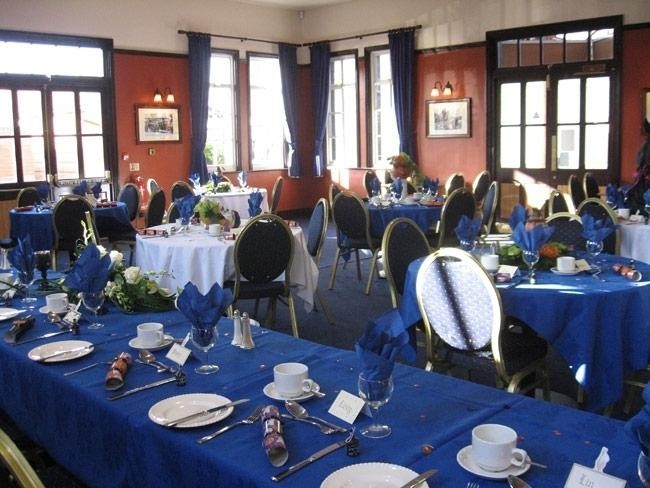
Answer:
[
  {"left": 265, "top": 381, "right": 320, "bottom": 405},
  {"left": 26, "top": 341, "right": 97, "bottom": 363},
  {"left": 148, "top": 392, "right": 233, "bottom": 429},
  {"left": 127, "top": 335, "right": 176, "bottom": 351},
  {"left": 320, "top": 461, "right": 431, "bottom": 488},
  {"left": 39, "top": 304, "right": 76, "bottom": 314},
  {"left": 457, "top": 446, "right": 531, "bottom": 480}
]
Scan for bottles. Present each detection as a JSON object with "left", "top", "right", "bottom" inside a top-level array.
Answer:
[
  {"left": 241, "top": 312, "right": 255, "bottom": 352},
  {"left": 72, "top": 318, "right": 80, "bottom": 335},
  {"left": 231, "top": 310, "right": 243, "bottom": 348}
]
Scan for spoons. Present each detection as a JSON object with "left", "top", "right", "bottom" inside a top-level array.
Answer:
[
  {"left": 506, "top": 472, "right": 533, "bottom": 488},
  {"left": 139, "top": 349, "right": 178, "bottom": 375},
  {"left": 285, "top": 398, "right": 352, "bottom": 436},
  {"left": 48, "top": 312, "right": 70, "bottom": 331}
]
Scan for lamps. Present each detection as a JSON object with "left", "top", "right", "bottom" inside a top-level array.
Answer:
[
  {"left": 430, "top": 80, "right": 453, "bottom": 97},
  {"left": 152, "top": 86, "right": 177, "bottom": 104}
]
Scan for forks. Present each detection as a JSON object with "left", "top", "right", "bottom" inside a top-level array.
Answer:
[
  {"left": 135, "top": 359, "right": 168, "bottom": 374},
  {"left": 465, "top": 482, "right": 480, "bottom": 488},
  {"left": 200, "top": 405, "right": 263, "bottom": 445},
  {"left": 282, "top": 414, "right": 339, "bottom": 436}
]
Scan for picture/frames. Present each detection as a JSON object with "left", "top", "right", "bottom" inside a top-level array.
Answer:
[
  {"left": 425, "top": 98, "right": 472, "bottom": 139},
  {"left": 134, "top": 102, "right": 183, "bottom": 144}
]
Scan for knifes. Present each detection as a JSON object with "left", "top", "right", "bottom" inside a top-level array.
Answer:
[
  {"left": 166, "top": 399, "right": 249, "bottom": 428},
  {"left": 270, "top": 441, "right": 347, "bottom": 481},
  {"left": 35, "top": 344, "right": 94, "bottom": 360},
  {"left": 398, "top": 469, "right": 438, "bottom": 488},
  {"left": 107, "top": 376, "right": 173, "bottom": 403},
  {"left": 14, "top": 330, "right": 66, "bottom": 345}
]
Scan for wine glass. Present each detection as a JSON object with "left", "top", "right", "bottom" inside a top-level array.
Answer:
[
  {"left": 190, "top": 327, "right": 221, "bottom": 374},
  {"left": 608, "top": 197, "right": 649, "bottom": 222},
  {"left": 372, "top": 184, "right": 441, "bottom": 208},
  {"left": 0, "top": 247, "right": 37, "bottom": 322},
  {"left": 356, "top": 374, "right": 395, "bottom": 439},
  {"left": 465, "top": 239, "right": 603, "bottom": 284},
  {"left": 191, "top": 179, "right": 249, "bottom": 192},
  {"left": 82, "top": 293, "right": 107, "bottom": 329}
]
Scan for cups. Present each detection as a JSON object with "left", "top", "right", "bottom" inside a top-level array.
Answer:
[
  {"left": 138, "top": 323, "right": 165, "bottom": 345},
  {"left": 165, "top": 210, "right": 239, "bottom": 237},
  {"left": 273, "top": 363, "right": 313, "bottom": 396},
  {"left": 46, "top": 293, "right": 69, "bottom": 312},
  {"left": 473, "top": 422, "right": 529, "bottom": 473}
]
